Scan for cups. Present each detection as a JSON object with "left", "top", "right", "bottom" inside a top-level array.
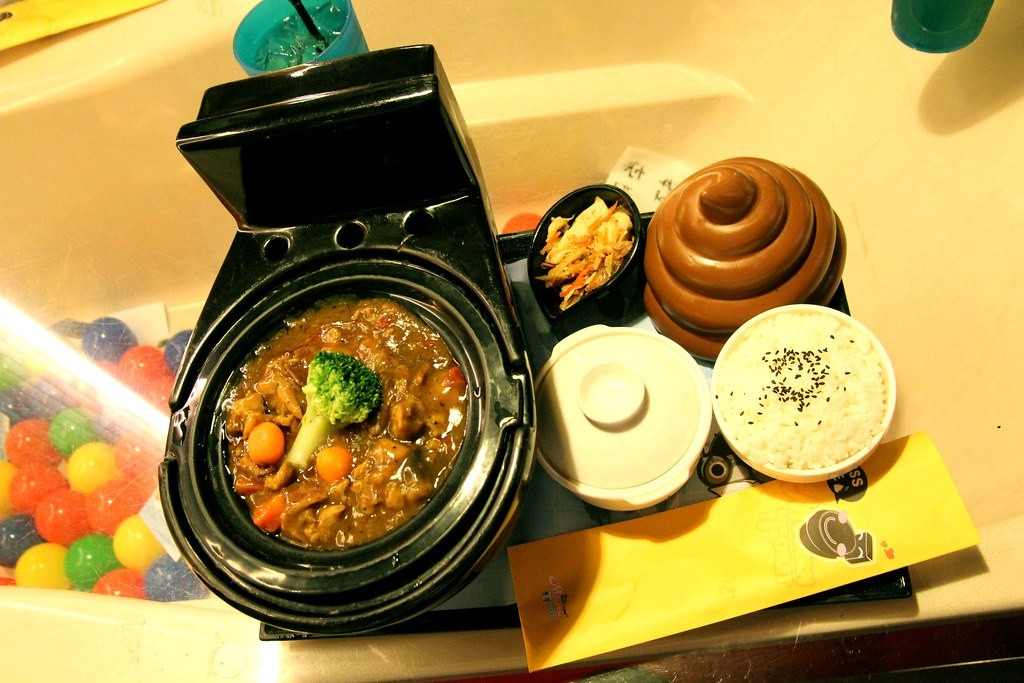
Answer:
[{"left": 232, "top": 0, "right": 371, "bottom": 78}]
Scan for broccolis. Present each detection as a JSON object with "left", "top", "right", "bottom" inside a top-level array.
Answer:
[{"left": 284, "top": 351, "right": 381, "bottom": 471}]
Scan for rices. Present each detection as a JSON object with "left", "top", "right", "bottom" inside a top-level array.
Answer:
[{"left": 716, "top": 311, "right": 888, "bottom": 470}]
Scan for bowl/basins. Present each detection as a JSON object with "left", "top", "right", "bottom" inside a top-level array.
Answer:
[
  {"left": 527, "top": 182, "right": 645, "bottom": 336},
  {"left": 529, "top": 324, "right": 714, "bottom": 511},
  {"left": 711, "top": 303, "right": 898, "bottom": 485}
]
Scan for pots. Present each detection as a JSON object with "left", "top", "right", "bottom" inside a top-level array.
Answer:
[{"left": 157, "top": 44, "right": 534, "bottom": 636}]
